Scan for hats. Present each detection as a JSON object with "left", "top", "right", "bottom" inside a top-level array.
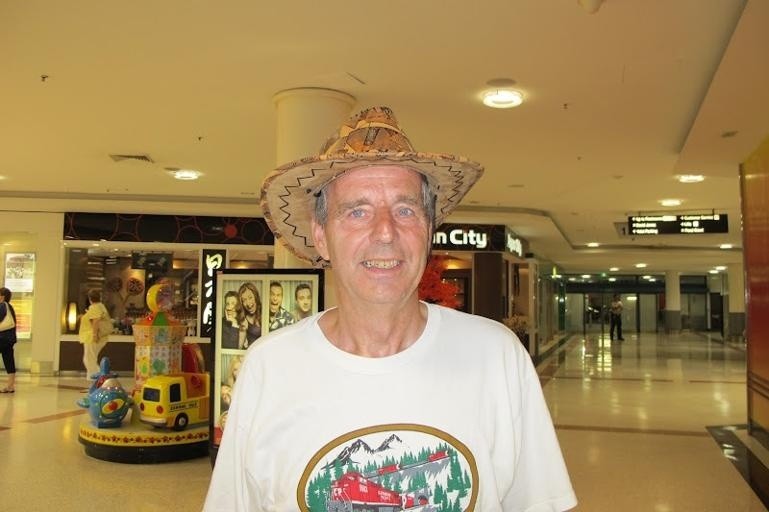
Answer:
[{"left": 255, "top": 104, "right": 485, "bottom": 270}]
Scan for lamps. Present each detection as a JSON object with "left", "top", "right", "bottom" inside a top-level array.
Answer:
[
  {"left": 479, "top": 88, "right": 525, "bottom": 110},
  {"left": 66, "top": 300, "right": 79, "bottom": 334}
]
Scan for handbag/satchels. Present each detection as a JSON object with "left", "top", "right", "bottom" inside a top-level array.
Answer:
[
  {"left": 97, "top": 313, "right": 115, "bottom": 337},
  {"left": 0, "top": 301, "right": 17, "bottom": 332}
]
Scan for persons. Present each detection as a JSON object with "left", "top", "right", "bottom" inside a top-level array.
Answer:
[
  {"left": 200, "top": 106, "right": 578, "bottom": 511},
  {"left": 0, "top": 287, "right": 17, "bottom": 393},
  {"left": 78, "top": 289, "right": 112, "bottom": 381},
  {"left": 221, "top": 282, "right": 313, "bottom": 415},
  {"left": 609, "top": 293, "right": 624, "bottom": 341}
]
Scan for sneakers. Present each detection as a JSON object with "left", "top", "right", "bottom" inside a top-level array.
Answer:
[
  {"left": 609, "top": 335, "right": 626, "bottom": 343},
  {"left": 0, "top": 386, "right": 16, "bottom": 394}
]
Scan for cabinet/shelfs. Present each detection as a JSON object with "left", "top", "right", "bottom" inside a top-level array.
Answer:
[{"left": 436, "top": 275, "right": 468, "bottom": 312}]
